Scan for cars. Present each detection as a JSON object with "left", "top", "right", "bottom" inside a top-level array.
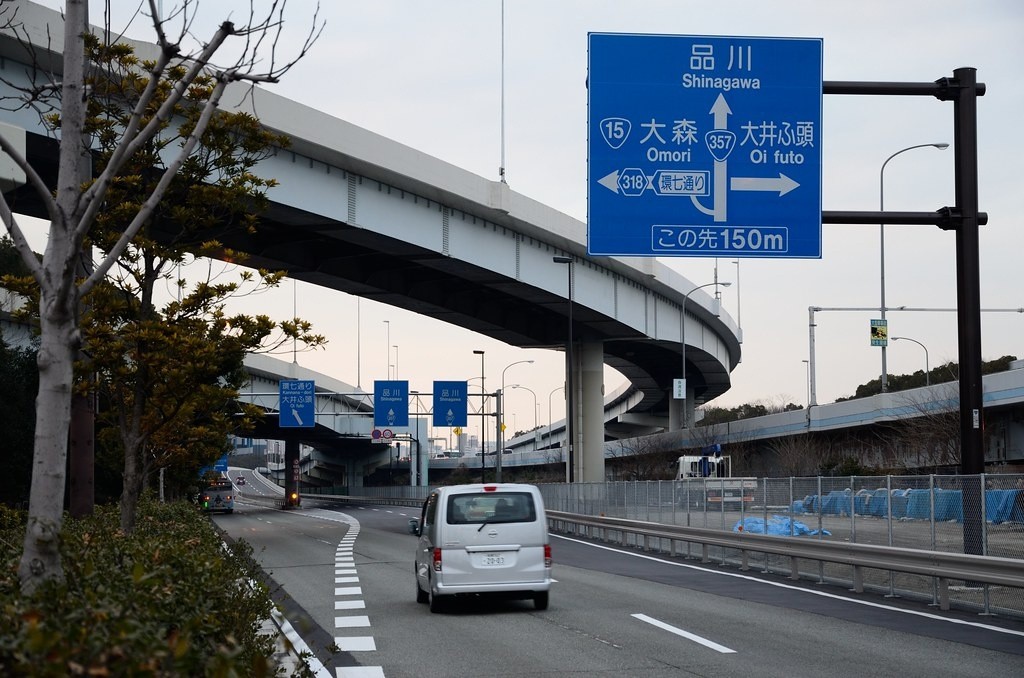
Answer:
[{"left": 236, "top": 476, "right": 246, "bottom": 485}]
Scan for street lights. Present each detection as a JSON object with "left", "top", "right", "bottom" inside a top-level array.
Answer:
[
  {"left": 891, "top": 337, "right": 929, "bottom": 387},
  {"left": 879, "top": 143, "right": 950, "bottom": 395},
  {"left": 680, "top": 282, "right": 732, "bottom": 430},
  {"left": 392, "top": 345, "right": 398, "bottom": 381},
  {"left": 382, "top": 320, "right": 389, "bottom": 381},
  {"left": 503, "top": 360, "right": 535, "bottom": 454},
  {"left": 474, "top": 350, "right": 485, "bottom": 484},
  {"left": 511, "top": 386, "right": 537, "bottom": 452},
  {"left": 552, "top": 256, "right": 574, "bottom": 485}
]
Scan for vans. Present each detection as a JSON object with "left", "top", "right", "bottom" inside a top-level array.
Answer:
[{"left": 409, "top": 481, "right": 559, "bottom": 614}]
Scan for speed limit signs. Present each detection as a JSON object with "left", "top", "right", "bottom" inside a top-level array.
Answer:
[{"left": 383, "top": 429, "right": 393, "bottom": 439}]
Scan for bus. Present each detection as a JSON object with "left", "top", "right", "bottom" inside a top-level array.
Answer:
[{"left": 198, "top": 470, "right": 234, "bottom": 514}]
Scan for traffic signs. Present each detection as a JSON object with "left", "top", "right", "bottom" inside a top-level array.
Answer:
[
  {"left": 585, "top": 32, "right": 824, "bottom": 260},
  {"left": 279, "top": 380, "right": 316, "bottom": 428},
  {"left": 433, "top": 380, "right": 468, "bottom": 427},
  {"left": 373, "top": 380, "right": 410, "bottom": 428},
  {"left": 199, "top": 454, "right": 228, "bottom": 476}
]
